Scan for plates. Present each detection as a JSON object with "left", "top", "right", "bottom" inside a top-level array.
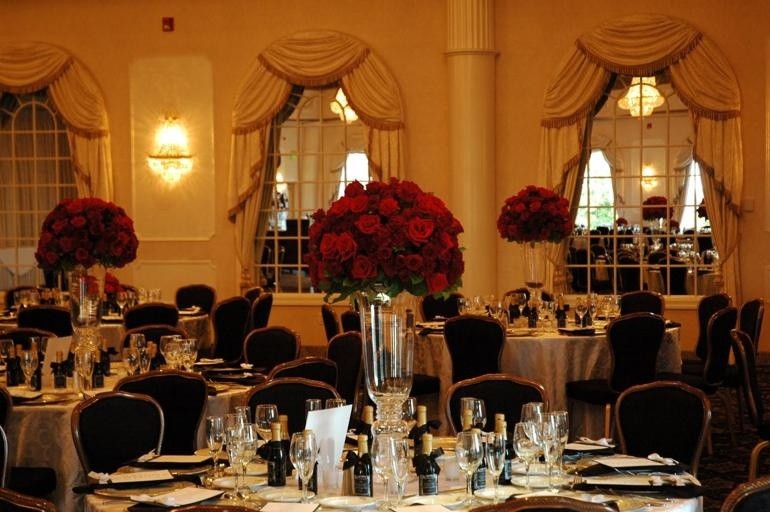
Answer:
[
  {"left": 136, "top": 465, "right": 211, "bottom": 479},
  {"left": 426, "top": 493, "right": 466, "bottom": 506},
  {"left": 258, "top": 489, "right": 315, "bottom": 501},
  {"left": 319, "top": 496, "right": 376, "bottom": 508},
  {"left": 225, "top": 461, "right": 268, "bottom": 475},
  {"left": 195, "top": 360, "right": 224, "bottom": 365},
  {"left": 474, "top": 488, "right": 513, "bottom": 499},
  {"left": 96, "top": 482, "right": 195, "bottom": 498},
  {"left": 511, "top": 476, "right": 564, "bottom": 488},
  {"left": 196, "top": 447, "right": 228, "bottom": 459},
  {"left": 214, "top": 475, "right": 267, "bottom": 489}
]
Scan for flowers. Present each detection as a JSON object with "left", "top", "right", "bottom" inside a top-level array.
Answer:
[
  {"left": 33, "top": 196, "right": 139, "bottom": 278},
  {"left": 497, "top": 184, "right": 577, "bottom": 243},
  {"left": 695, "top": 197, "right": 709, "bottom": 221},
  {"left": 640, "top": 195, "right": 675, "bottom": 220},
  {"left": 299, "top": 173, "right": 470, "bottom": 309}
]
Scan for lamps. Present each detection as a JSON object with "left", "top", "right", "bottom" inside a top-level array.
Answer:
[
  {"left": 146, "top": 145, "right": 195, "bottom": 185},
  {"left": 616, "top": 75, "right": 665, "bottom": 119},
  {"left": 328, "top": 87, "right": 360, "bottom": 126}
]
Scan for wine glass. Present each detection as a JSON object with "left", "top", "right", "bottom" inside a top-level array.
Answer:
[
  {"left": 289, "top": 431, "right": 308, "bottom": 497},
  {"left": 159, "top": 334, "right": 199, "bottom": 373},
  {"left": 454, "top": 290, "right": 622, "bottom": 336},
  {"left": 539, "top": 413, "right": 562, "bottom": 496},
  {"left": 138, "top": 348, "right": 151, "bottom": 375},
  {"left": 295, "top": 435, "right": 320, "bottom": 506},
  {"left": 549, "top": 410, "right": 571, "bottom": 485},
  {"left": 389, "top": 437, "right": 410, "bottom": 507},
  {"left": 234, "top": 423, "right": 258, "bottom": 500},
  {"left": 521, "top": 403, "right": 543, "bottom": 475},
  {"left": 255, "top": 403, "right": 282, "bottom": 464},
  {"left": 203, "top": 415, "right": 226, "bottom": 489},
  {"left": 19, "top": 350, "right": 38, "bottom": 391},
  {"left": 485, "top": 432, "right": 506, "bottom": 500},
  {"left": 455, "top": 431, "right": 484, "bottom": 506},
  {"left": 224, "top": 427, "right": 244, "bottom": 501},
  {"left": 610, "top": 216, "right": 713, "bottom": 264},
  {"left": 403, "top": 396, "right": 417, "bottom": 420},
  {"left": 116, "top": 287, "right": 162, "bottom": 319},
  {"left": 371, "top": 434, "right": 391, "bottom": 511},
  {"left": 513, "top": 422, "right": 543, "bottom": 494},
  {"left": 74, "top": 348, "right": 93, "bottom": 401},
  {"left": 122, "top": 347, "right": 140, "bottom": 375}
]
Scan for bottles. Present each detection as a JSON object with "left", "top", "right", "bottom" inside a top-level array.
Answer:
[
  {"left": 267, "top": 422, "right": 286, "bottom": 487},
  {"left": 412, "top": 406, "right": 430, "bottom": 466},
  {"left": 51, "top": 351, "right": 66, "bottom": 389},
  {"left": 99, "top": 339, "right": 110, "bottom": 376},
  {"left": 92, "top": 350, "right": 104, "bottom": 389},
  {"left": 15, "top": 343, "right": 26, "bottom": 383},
  {"left": 493, "top": 420, "right": 512, "bottom": 485},
  {"left": 360, "top": 405, "right": 375, "bottom": 448},
  {"left": 3, "top": 339, "right": 21, "bottom": 386},
  {"left": 28, "top": 336, "right": 44, "bottom": 391},
  {"left": 419, "top": 434, "right": 439, "bottom": 496},
  {"left": 492, "top": 413, "right": 510, "bottom": 445},
  {"left": 354, "top": 434, "right": 372, "bottom": 496},
  {"left": 465, "top": 427, "right": 486, "bottom": 494},
  {"left": 278, "top": 414, "right": 292, "bottom": 476}
]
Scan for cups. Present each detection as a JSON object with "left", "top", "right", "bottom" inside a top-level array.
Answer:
[
  {"left": 465, "top": 399, "right": 487, "bottom": 429},
  {"left": 325, "top": 398, "right": 347, "bottom": 410},
  {"left": 460, "top": 397, "right": 476, "bottom": 427},
  {"left": 13, "top": 289, "right": 38, "bottom": 318},
  {"left": 226, "top": 413, "right": 248, "bottom": 440},
  {"left": 304, "top": 398, "right": 321, "bottom": 416},
  {"left": 234, "top": 405, "right": 252, "bottom": 426},
  {"left": 129, "top": 333, "right": 146, "bottom": 356}
]
